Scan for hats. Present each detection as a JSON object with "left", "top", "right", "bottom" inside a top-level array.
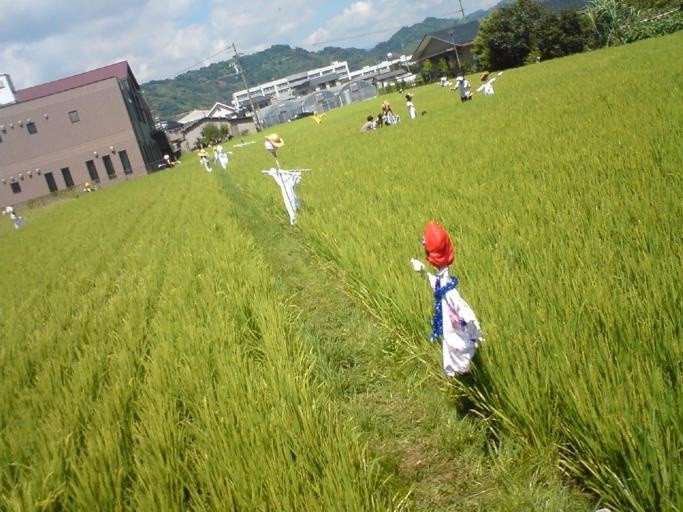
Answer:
[
  {"left": 164, "top": 155, "right": 169, "bottom": 159},
  {"left": 265, "top": 133, "right": 284, "bottom": 147},
  {"left": 84, "top": 183, "right": 89, "bottom": 187},
  {"left": 423, "top": 222, "right": 454, "bottom": 267},
  {"left": 198, "top": 149, "right": 209, "bottom": 157},
  {"left": 6, "top": 207, "right": 13, "bottom": 214},
  {"left": 479, "top": 71, "right": 488, "bottom": 81},
  {"left": 456, "top": 76, "right": 464, "bottom": 81}
]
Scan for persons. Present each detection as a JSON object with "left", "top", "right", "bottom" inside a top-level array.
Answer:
[
  {"left": 260, "top": 134, "right": 313, "bottom": 227},
  {"left": 439, "top": 68, "right": 503, "bottom": 103},
  {"left": 5, "top": 205, "right": 26, "bottom": 229},
  {"left": 159, "top": 130, "right": 233, "bottom": 174},
  {"left": 82, "top": 181, "right": 97, "bottom": 194},
  {"left": 409, "top": 219, "right": 483, "bottom": 417},
  {"left": 358, "top": 87, "right": 415, "bottom": 133},
  {"left": 313, "top": 108, "right": 328, "bottom": 126}
]
